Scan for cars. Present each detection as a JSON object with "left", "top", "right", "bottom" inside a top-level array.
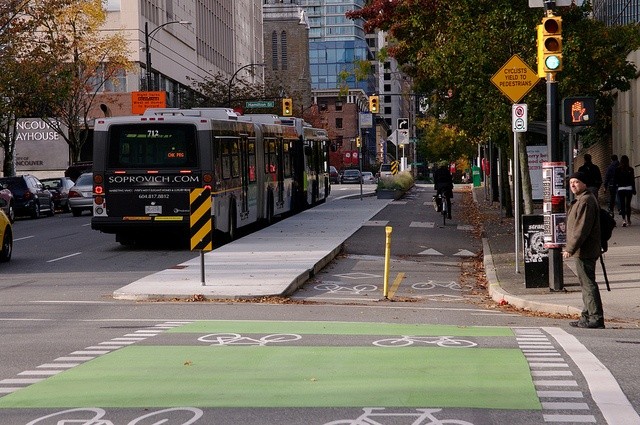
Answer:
[
  {"left": 0, "top": 174, "right": 55, "bottom": 219},
  {"left": 68, "top": 172, "right": 94, "bottom": 217},
  {"left": 330, "top": 166, "right": 339, "bottom": 184},
  {"left": 39, "top": 177, "right": 76, "bottom": 213},
  {"left": 0, "top": 208, "right": 13, "bottom": 263},
  {"left": 341, "top": 169, "right": 361, "bottom": 184},
  {"left": 362, "top": 172, "right": 374, "bottom": 184},
  {"left": 0, "top": 182, "right": 15, "bottom": 225}
]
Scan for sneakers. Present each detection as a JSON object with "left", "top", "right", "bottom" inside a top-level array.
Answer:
[
  {"left": 623, "top": 222, "right": 626, "bottom": 227},
  {"left": 627, "top": 221, "right": 631, "bottom": 226},
  {"left": 585, "top": 321, "right": 604, "bottom": 328},
  {"left": 569, "top": 321, "right": 585, "bottom": 327}
]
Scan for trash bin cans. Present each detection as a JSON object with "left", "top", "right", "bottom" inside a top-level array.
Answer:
[{"left": 471, "top": 165, "right": 481, "bottom": 187}]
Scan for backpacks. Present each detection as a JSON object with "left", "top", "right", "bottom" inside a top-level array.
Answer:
[{"left": 599, "top": 208, "right": 616, "bottom": 252}]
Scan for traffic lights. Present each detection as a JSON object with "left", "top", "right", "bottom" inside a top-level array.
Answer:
[
  {"left": 369, "top": 96, "right": 380, "bottom": 114},
  {"left": 563, "top": 96, "right": 593, "bottom": 127},
  {"left": 356, "top": 138, "right": 361, "bottom": 147},
  {"left": 543, "top": 16, "right": 562, "bottom": 73},
  {"left": 283, "top": 99, "right": 292, "bottom": 116}
]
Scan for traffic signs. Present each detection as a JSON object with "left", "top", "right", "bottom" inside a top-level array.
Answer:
[
  {"left": 359, "top": 112, "right": 373, "bottom": 129},
  {"left": 396, "top": 118, "right": 409, "bottom": 136},
  {"left": 245, "top": 101, "right": 275, "bottom": 109},
  {"left": 489, "top": 53, "right": 540, "bottom": 103}
]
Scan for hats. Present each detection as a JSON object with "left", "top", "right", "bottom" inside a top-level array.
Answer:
[
  {"left": 439, "top": 161, "right": 448, "bottom": 166},
  {"left": 570, "top": 172, "right": 587, "bottom": 184}
]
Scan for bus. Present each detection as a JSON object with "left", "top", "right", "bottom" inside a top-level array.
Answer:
[{"left": 91, "top": 107, "right": 331, "bottom": 242}]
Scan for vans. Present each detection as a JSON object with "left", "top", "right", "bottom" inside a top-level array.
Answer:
[{"left": 379, "top": 164, "right": 392, "bottom": 178}]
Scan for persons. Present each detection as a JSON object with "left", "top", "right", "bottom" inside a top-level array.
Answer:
[
  {"left": 577, "top": 153, "right": 602, "bottom": 200},
  {"left": 614, "top": 154, "right": 637, "bottom": 226},
  {"left": 557, "top": 220, "right": 566, "bottom": 241},
  {"left": 433, "top": 158, "right": 454, "bottom": 219},
  {"left": 603, "top": 154, "right": 619, "bottom": 218},
  {"left": 560, "top": 171, "right": 607, "bottom": 329}
]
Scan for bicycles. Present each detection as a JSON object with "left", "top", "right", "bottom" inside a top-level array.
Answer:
[{"left": 432, "top": 193, "right": 449, "bottom": 226}]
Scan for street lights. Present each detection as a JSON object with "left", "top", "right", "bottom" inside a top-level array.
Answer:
[
  {"left": 227, "top": 62, "right": 269, "bottom": 108},
  {"left": 140, "top": 20, "right": 193, "bottom": 91}
]
